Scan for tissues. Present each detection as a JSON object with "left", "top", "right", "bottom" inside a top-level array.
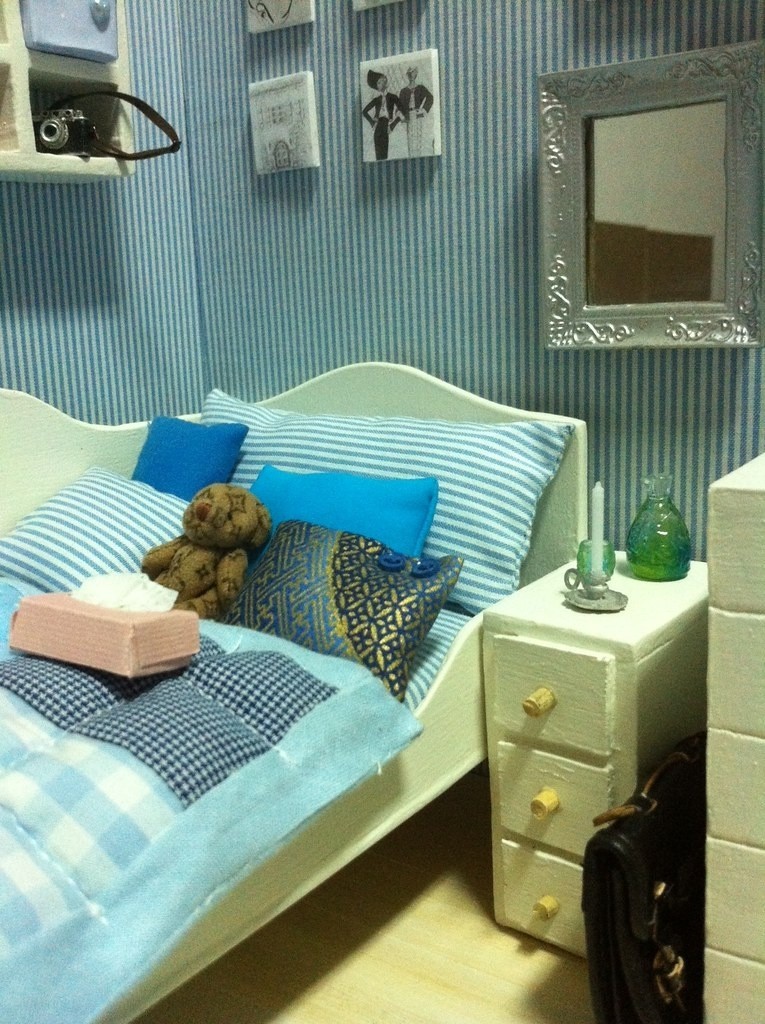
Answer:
[{"left": 7, "top": 571, "right": 201, "bottom": 681}]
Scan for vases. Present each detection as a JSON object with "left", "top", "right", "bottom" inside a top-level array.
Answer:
[{"left": 625, "top": 473, "right": 691, "bottom": 581}]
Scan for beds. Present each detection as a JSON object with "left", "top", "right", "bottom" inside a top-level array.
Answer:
[{"left": 0, "top": 361, "right": 588, "bottom": 1024}]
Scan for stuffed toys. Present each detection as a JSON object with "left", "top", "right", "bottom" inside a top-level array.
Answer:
[{"left": 141, "top": 483, "right": 272, "bottom": 618}]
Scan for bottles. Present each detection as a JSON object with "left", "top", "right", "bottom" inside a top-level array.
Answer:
[{"left": 625, "top": 475, "right": 691, "bottom": 582}]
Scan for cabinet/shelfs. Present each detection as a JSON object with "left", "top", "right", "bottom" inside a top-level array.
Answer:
[{"left": 0, "top": 0, "right": 136, "bottom": 184}]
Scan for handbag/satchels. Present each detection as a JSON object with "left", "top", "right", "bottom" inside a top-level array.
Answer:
[{"left": 581, "top": 731, "right": 707, "bottom": 1024}]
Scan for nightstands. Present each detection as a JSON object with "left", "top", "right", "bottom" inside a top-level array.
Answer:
[{"left": 483, "top": 549, "right": 709, "bottom": 960}]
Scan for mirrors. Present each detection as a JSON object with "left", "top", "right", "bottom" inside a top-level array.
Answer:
[{"left": 535, "top": 40, "right": 765, "bottom": 351}]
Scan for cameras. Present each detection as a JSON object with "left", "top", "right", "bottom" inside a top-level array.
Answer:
[{"left": 31, "top": 108, "right": 92, "bottom": 157}]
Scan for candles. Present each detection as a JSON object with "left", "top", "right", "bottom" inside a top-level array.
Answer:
[{"left": 591, "top": 481, "right": 604, "bottom": 573}]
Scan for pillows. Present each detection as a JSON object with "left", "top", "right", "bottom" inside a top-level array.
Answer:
[
  {"left": 0, "top": 465, "right": 190, "bottom": 592},
  {"left": 132, "top": 415, "right": 249, "bottom": 501},
  {"left": 245, "top": 464, "right": 439, "bottom": 575},
  {"left": 199, "top": 388, "right": 577, "bottom": 615},
  {"left": 221, "top": 519, "right": 465, "bottom": 701}
]
